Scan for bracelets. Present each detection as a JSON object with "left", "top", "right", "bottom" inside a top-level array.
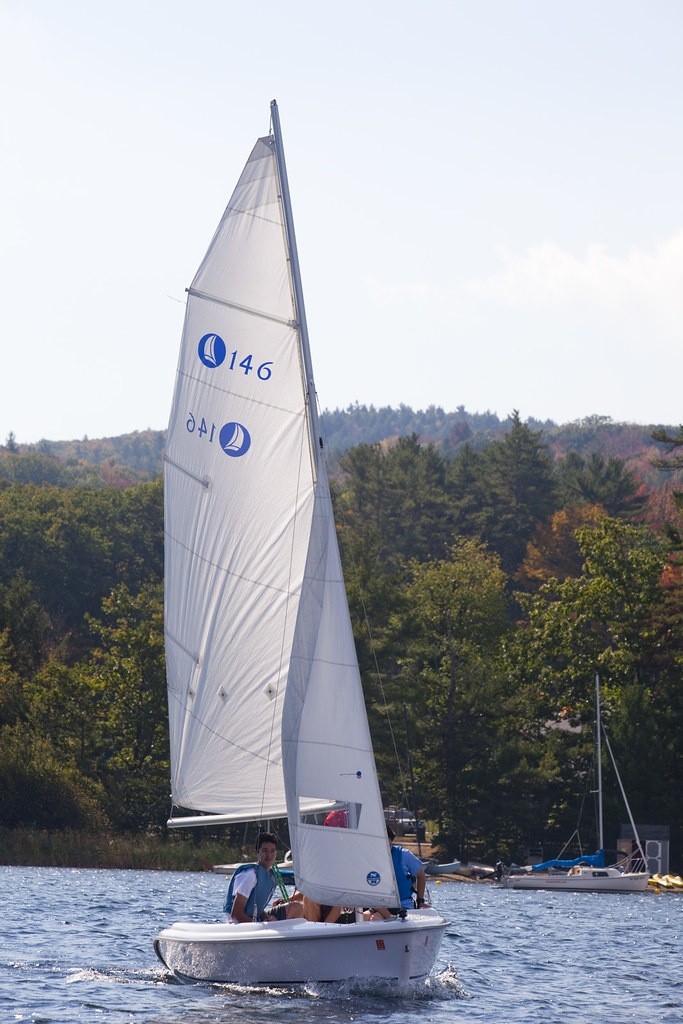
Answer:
[{"left": 416, "top": 897, "right": 425, "bottom": 904}]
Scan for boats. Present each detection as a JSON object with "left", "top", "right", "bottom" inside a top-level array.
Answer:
[
  {"left": 648, "top": 873, "right": 683, "bottom": 888},
  {"left": 418, "top": 858, "right": 495, "bottom": 879}
]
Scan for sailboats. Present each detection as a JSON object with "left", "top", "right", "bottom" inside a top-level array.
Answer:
[
  {"left": 153, "top": 98, "right": 452, "bottom": 1001},
  {"left": 503, "top": 674, "right": 650, "bottom": 891}
]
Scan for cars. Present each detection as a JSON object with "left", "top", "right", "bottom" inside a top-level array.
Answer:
[{"left": 383, "top": 805, "right": 426, "bottom": 837}]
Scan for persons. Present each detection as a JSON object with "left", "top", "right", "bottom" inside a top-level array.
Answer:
[
  {"left": 495, "top": 860, "right": 504, "bottom": 883},
  {"left": 223, "top": 833, "right": 303, "bottom": 923},
  {"left": 273, "top": 893, "right": 340, "bottom": 923},
  {"left": 364, "top": 825, "right": 430, "bottom": 922}
]
